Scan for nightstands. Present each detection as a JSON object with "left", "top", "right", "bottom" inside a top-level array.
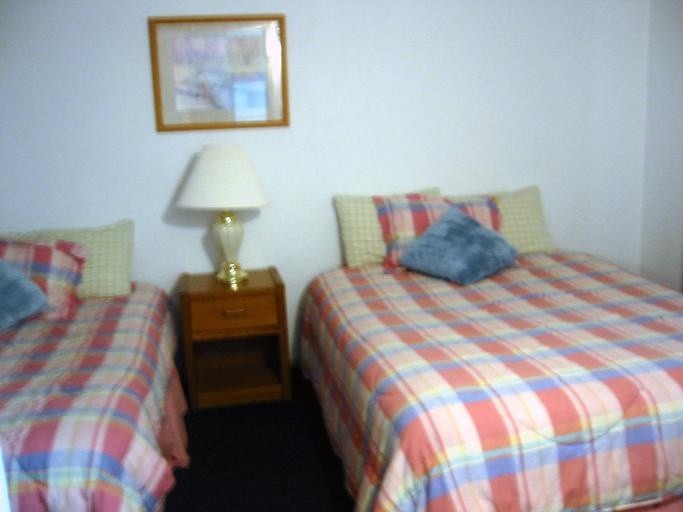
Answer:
[{"left": 176, "top": 267, "right": 291, "bottom": 414}]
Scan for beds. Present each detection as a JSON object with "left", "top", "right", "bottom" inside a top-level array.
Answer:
[
  {"left": 0, "top": 280, "right": 192, "bottom": 512},
  {"left": 295, "top": 250, "right": 683, "bottom": 512}
]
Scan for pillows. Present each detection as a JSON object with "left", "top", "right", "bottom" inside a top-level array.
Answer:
[
  {"left": 0, "top": 217, "right": 133, "bottom": 338},
  {"left": 331, "top": 184, "right": 560, "bottom": 286}
]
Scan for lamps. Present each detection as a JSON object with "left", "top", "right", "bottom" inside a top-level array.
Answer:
[{"left": 173, "top": 142, "right": 270, "bottom": 285}]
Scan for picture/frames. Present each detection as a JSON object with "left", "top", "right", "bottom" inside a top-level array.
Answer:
[{"left": 147, "top": 13, "right": 290, "bottom": 132}]
